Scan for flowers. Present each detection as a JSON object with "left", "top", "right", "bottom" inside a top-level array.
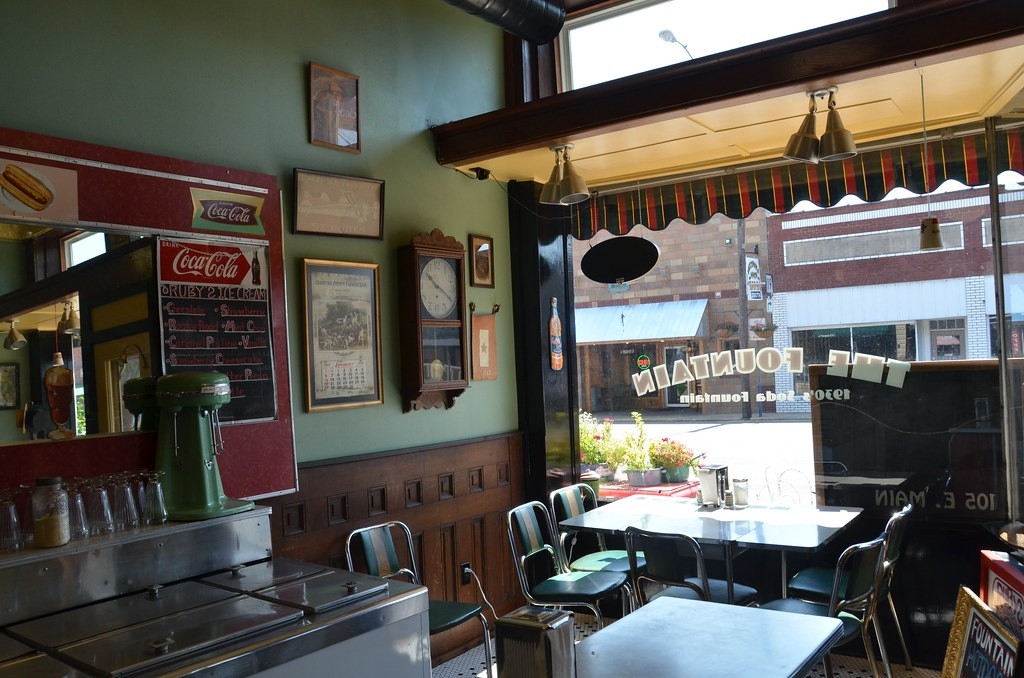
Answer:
[
  {"left": 715, "top": 320, "right": 738, "bottom": 332},
  {"left": 577, "top": 406, "right": 705, "bottom": 476},
  {"left": 750, "top": 320, "right": 778, "bottom": 333}
]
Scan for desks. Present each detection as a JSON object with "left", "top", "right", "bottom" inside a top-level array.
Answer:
[
  {"left": 559, "top": 494, "right": 867, "bottom": 600},
  {"left": 573, "top": 596, "right": 844, "bottom": 678}
]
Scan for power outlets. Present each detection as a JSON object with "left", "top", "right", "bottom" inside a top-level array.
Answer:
[{"left": 461, "top": 562, "right": 471, "bottom": 586}]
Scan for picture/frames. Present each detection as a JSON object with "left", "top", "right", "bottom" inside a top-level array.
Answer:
[
  {"left": 307, "top": 61, "right": 361, "bottom": 154},
  {"left": 104, "top": 351, "right": 151, "bottom": 433},
  {"left": 301, "top": 258, "right": 384, "bottom": 413},
  {"left": 0, "top": 361, "right": 21, "bottom": 409},
  {"left": 941, "top": 585, "right": 1021, "bottom": 678},
  {"left": 467, "top": 233, "right": 495, "bottom": 288},
  {"left": 292, "top": 167, "right": 386, "bottom": 240}
]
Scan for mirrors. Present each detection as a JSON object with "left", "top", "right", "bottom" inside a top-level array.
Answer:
[{"left": 0, "top": 217, "right": 166, "bottom": 447}]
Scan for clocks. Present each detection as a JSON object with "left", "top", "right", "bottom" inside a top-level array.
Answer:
[{"left": 396, "top": 229, "right": 470, "bottom": 415}]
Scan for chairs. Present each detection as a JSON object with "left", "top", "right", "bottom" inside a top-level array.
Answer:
[{"left": 345, "top": 487, "right": 912, "bottom": 678}]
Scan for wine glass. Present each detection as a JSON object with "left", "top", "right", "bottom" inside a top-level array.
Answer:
[
  {"left": 0, "top": 484, "right": 35, "bottom": 555},
  {"left": 109, "top": 471, "right": 139, "bottom": 532},
  {"left": 62, "top": 477, "right": 89, "bottom": 541},
  {"left": 85, "top": 474, "right": 115, "bottom": 536},
  {"left": 140, "top": 470, "right": 169, "bottom": 525}
]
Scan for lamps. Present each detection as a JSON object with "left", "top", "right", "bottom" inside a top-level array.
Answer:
[
  {"left": 781, "top": 85, "right": 858, "bottom": 166},
  {"left": 539, "top": 144, "right": 590, "bottom": 206},
  {"left": 658, "top": 29, "right": 693, "bottom": 61},
  {"left": 57, "top": 300, "right": 81, "bottom": 334},
  {"left": 3, "top": 320, "right": 27, "bottom": 350},
  {"left": 919, "top": 68, "right": 944, "bottom": 251}
]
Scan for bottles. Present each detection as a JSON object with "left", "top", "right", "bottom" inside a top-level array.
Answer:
[
  {"left": 550, "top": 296, "right": 563, "bottom": 370},
  {"left": 697, "top": 490, "right": 703, "bottom": 503},
  {"left": 31, "top": 476, "right": 71, "bottom": 549},
  {"left": 733, "top": 479, "right": 749, "bottom": 509},
  {"left": 723, "top": 490, "right": 734, "bottom": 509},
  {"left": 252, "top": 251, "right": 261, "bottom": 284}
]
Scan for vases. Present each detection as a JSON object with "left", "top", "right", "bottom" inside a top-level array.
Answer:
[
  {"left": 665, "top": 464, "right": 690, "bottom": 483},
  {"left": 755, "top": 330, "right": 774, "bottom": 338},
  {"left": 581, "top": 464, "right": 617, "bottom": 481},
  {"left": 624, "top": 468, "right": 661, "bottom": 488},
  {"left": 716, "top": 328, "right": 735, "bottom": 338}
]
